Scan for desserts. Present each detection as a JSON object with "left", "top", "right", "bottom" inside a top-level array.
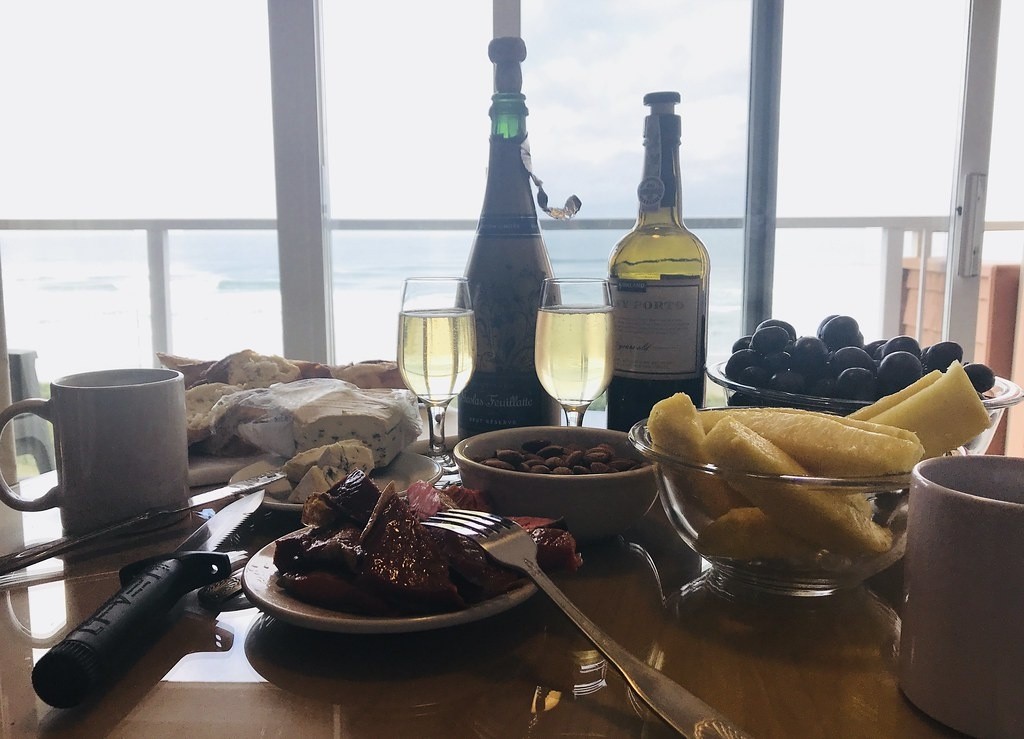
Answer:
[{"left": 157, "top": 350, "right": 420, "bottom": 498}]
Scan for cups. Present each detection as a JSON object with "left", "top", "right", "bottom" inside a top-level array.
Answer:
[
  {"left": 0, "top": 367, "right": 191, "bottom": 540},
  {"left": 896, "top": 455, "right": 1024, "bottom": 739}
]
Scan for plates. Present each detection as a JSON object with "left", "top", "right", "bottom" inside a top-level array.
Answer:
[
  {"left": 242, "top": 524, "right": 543, "bottom": 634},
  {"left": 228, "top": 450, "right": 443, "bottom": 510},
  {"left": 246, "top": 612, "right": 536, "bottom": 703}
]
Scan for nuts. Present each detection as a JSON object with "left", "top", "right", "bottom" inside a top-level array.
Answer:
[{"left": 474, "top": 441, "right": 647, "bottom": 475}]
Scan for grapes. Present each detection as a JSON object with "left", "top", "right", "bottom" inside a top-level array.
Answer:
[{"left": 726, "top": 314, "right": 995, "bottom": 402}]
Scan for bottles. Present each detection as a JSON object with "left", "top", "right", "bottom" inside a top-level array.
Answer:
[
  {"left": 455, "top": 93, "right": 563, "bottom": 444},
  {"left": 605, "top": 113, "right": 711, "bottom": 433}
]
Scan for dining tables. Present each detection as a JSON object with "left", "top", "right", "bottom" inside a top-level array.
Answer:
[{"left": 0, "top": 410, "right": 965, "bottom": 739}]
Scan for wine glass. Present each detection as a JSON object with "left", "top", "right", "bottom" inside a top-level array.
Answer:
[
  {"left": 396, "top": 276, "right": 478, "bottom": 486},
  {"left": 533, "top": 277, "right": 617, "bottom": 428}
]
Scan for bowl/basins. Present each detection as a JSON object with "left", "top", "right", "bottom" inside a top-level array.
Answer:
[
  {"left": 453, "top": 426, "right": 661, "bottom": 546},
  {"left": 708, "top": 357, "right": 1024, "bottom": 456},
  {"left": 629, "top": 405, "right": 971, "bottom": 598}
]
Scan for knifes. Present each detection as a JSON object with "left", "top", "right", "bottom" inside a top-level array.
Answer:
[
  {"left": 0, "top": 471, "right": 287, "bottom": 576},
  {"left": 31, "top": 488, "right": 266, "bottom": 709}
]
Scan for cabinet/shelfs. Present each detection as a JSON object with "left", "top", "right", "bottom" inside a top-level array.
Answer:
[{"left": 900, "top": 256, "right": 1020, "bottom": 457}]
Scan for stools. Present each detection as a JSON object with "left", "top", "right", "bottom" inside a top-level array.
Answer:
[{"left": 8, "top": 349, "right": 55, "bottom": 481}]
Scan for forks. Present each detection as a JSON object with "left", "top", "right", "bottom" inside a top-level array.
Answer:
[{"left": 421, "top": 506, "right": 754, "bottom": 739}]
